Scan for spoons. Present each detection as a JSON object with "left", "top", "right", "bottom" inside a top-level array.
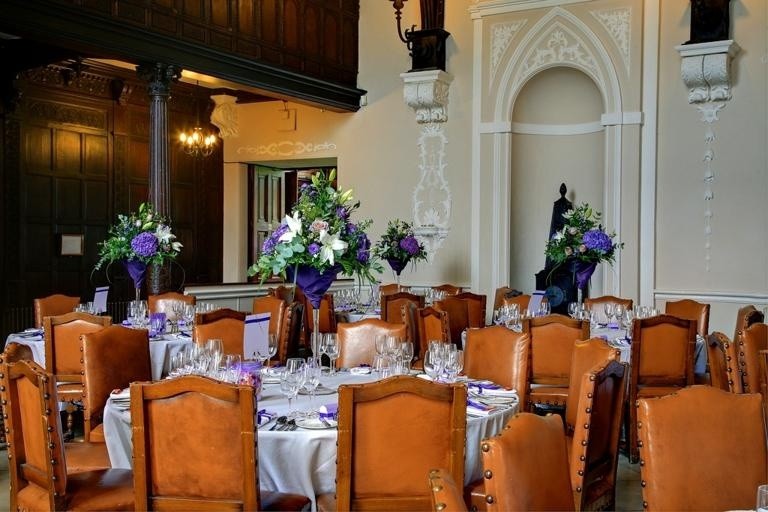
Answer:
[{"left": 269, "top": 415, "right": 288, "bottom": 431}]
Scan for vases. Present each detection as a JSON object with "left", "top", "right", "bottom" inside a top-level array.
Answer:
[
  {"left": 396, "top": 275, "right": 401, "bottom": 294},
  {"left": 298, "top": 309, "right": 337, "bottom": 395},
  {"left": 136, "top": 288, "right": 140, "bottom": 308},
  {"left": 577, "top": 287, "right": 582, "bottom": 308}
]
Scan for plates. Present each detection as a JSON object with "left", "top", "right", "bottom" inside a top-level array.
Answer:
[{"left": 295, "top": 418, "right": 338, "bottom": 429}]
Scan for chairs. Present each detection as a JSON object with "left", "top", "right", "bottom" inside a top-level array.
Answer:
[
  {"left": 266, "top": 301, "right": 306, "bottom": 368},
  {"left": 584, "top": 296, "right": 633, "bottom": 316},
  {"left": 0, "top": 343, "right": 112, "bottom": 474},
  {"left": 42, "top": 313, "right": 112, "bottom": 441},
  {"left": 431, "top": 284, "right": 463, "bottom": 299},
  {"left": 480, "top": 414, "right": 576, "bottom": 511},
  {"left": 666, "top": 299, "right": 710, "bottom": 338},
  {"left": 79, "top": 324, "right": 152, "bottom": 443},
  {"left": 434, "top": 298, "right": 469, "bottom": 351},
  {"left": 194, "top": 318, "right": 254, "bottom": 365},
  {"left": 705, "top": 330, "right": 742, "bottom": 394},
  {"left": 130, "top": 375, "right": 312, "bottom": 512},
  {"left": 380, "top": 292, "right": 424, "bottom": 324},
  {"left": 195, "top": 307, "right": 251, "bottom": 326},
  {"left": 462, "top": 324, "right": 529, "bottom": 416},
  {"left": 1, "top": 358, "right": 136, "bottom": 512},
  {"left": 492, "top": 287, "right": 531, "bottom": 324},
  {"left": 304, "top": 293, "right": 336, "bottom": 334},
  {"left": 275, "top": 285, "right": 304, "bottom": 306},
  {"left": 379, "top": 283, "right": 403, "bottom": 293},
  {"left": 737, "top": 323, "right": 768, "bottom": 435},
  {"left": 148, "top": 293, "right": 196, "bottom": 319},
  {"left": 34, "top": 294, "right": 81, "bottom": 329},
  {"left": 252, "top": 295, "right": 285, "bottom": 349},
  {"left": 335, "top": 318, "right": 407, "bottom": 370},
  {"left": 565, "top": 335, "right": 619, "bottom": 467},
  {"left": 463, "top": 359, "right": 631, "bottom": 512},
  {"left": 315, "top": 374, "right": 468, "bottom": 511},
  {"left": 635, "top": 385, "right": 768, "bottom": 512},
  {"left": 412, "top": 304, "right": 451, "bottom": 371},
  {"left": 427, "top": 467, "right": 469, "bottom": 512},
  {"left": 447, "top": 292, "right": 487, "bottom": 328},
  {"left": 619, "top": 314, "right": 698, "bottom": 463},
  {"left": 734, "top": 305, "right": 764, "bottom": 352},
  {"left": 400, "top": 300, "right": 417, "bottom": 354}
]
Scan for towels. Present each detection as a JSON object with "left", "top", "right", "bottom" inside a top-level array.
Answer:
[
  {"left": 349, "top": 363, "right": 371, "bottom": 375},
  {"left": 18, "top": 332, "right": 44, "bottom": 340},
  {"left": 318, "top": 404, "right": 339, "bottom": 420},
  {"left": 172, "top": 332, "right": 190, "bottom": 342},
  {"left": 468, "top": 382, "right": 517, "bottom": 397},
  {"left": 466, "top": 399, "right": 498, "bottom": 415},
  {"left": 256, "top": 409, "right": 277, "bottom": 429}
]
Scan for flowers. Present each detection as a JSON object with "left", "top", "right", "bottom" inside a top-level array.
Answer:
[
  {"left": 371, "top": 218, "right": 428, "bottom": 275},
  {"left": 90, "top": 202, "right": 186, "bottom": 292},
  {"left": 543, "top": 202, "right": 625, "bottom": 286},
  {"left": 248, "top": 168, "right": 386, "bottom": 321}
]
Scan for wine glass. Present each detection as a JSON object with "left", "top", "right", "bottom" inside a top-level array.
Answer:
[
  {"left": 171, "top": 299, "right": 220, "bottom": 338},
  {"left": 423, "top": 339, "right": 466, "bottom": 382},
  {"left": 402, "top": 286, "right": 446, "bottom": 306},
  {"left": 286, "top": 358, "right": 306, "bottom": 410},
  {"left": 322, "top": 333, "right": 342, "bottom": 377},
  {"left": 603, "top": 302, "right": 662, "bottom": 341},
  {"left": 370, "top": 334, "right": 414, "bottom": 379},
  {"left": 168, "top": 338, "right": 225, "bottom": 381},
  {"left": 126, "top": 300, "right": 164, "bottom": 340},
  {"left": 492, "top": 302, "right": 551, "bottom": 331},
  {"left": 303, "top": 357, "right": 322, "bottom": 408},
  {"left": 70, "top": 301, "right": 103, "bottom": 316},
  {"left": 310, "top": 332, "right": 327, "bottom": 368},
  {"left": 262, "top": 334, "right": 279, "bottom": 379},
  {"left": 226, "top": 354, "right": 242, "bottom": 385},
  {"left": 280, "top": 369, "right": 301, "bottom": 417},
  {"left": 568, "top": 302, "right": 597, "bottom": 328},
  {"left": 332, "top": 287, "right": 363, "bottom": 315}
]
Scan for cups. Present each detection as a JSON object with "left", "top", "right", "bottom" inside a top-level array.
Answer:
[{"left": 756, "top": 484, "right": 768, "bottom": 511}]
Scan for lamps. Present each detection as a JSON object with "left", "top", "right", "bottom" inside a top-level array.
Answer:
[{"left": 180, "top": 79, "right": 216, "bottom": 159}]
